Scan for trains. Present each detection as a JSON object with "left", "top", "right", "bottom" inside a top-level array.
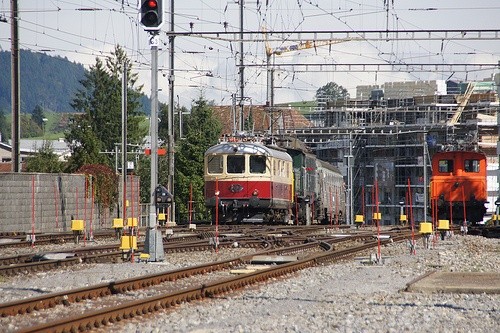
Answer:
[
  {"left": 429, "top": 152, "right": 487, "bottom": 225},
  {"left": 204, "top": 134, "right": 345, "bottom": 225}
]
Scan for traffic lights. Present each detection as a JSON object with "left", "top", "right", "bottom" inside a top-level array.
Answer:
[{"left": 140, "top": 0, "right": 162, "bottom": 27}]
